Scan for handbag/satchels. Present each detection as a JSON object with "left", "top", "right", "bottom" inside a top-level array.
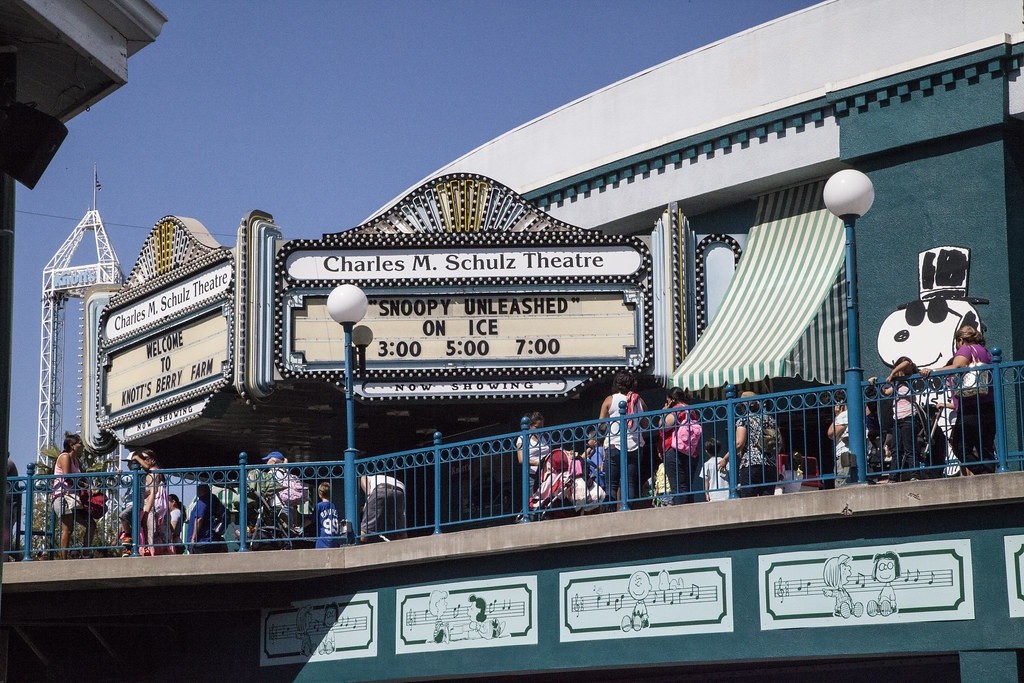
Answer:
[
  {"left": 64, "top": 492, "right": 83, "bottom": 511},
  {"left": 954, "top": 344, "right": 988, "bottom": 396},
  {"left": 781, "top": 465, "right": 802, "bottom": 493},
  {"left": 82, "top": 491, "right": 108, "bottom": 519}
]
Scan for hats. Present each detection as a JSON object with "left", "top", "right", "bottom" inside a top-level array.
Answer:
[
  {"left": 741, "top": 391, "right": 758, "bottom": 402},
  {"left": 261, "top": 452, "right": 284, "bottom": 461},
  {"left": 122, "top": 451, "right": 145, "bottom": 462}
]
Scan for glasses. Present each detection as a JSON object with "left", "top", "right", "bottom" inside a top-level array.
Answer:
[{"left": 534, "top": 425, "right": 541, "bottom": 429}]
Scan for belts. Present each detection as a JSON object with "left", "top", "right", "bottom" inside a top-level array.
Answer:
[{"left": 376, "top": 483, "right": 405, "bottom": 492}]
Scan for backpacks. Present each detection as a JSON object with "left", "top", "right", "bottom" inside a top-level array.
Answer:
[
  {"left": 750, "top": 416, "right": 780, "bottom": 454},
  {"left": 670, "top": 403, "right": 701, "bottom": 456},
  {"left": 208, "top": 500, "right": 229, "bottom": 537}
]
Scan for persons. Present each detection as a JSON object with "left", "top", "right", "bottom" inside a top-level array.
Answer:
[
  {"left": 93, "top": 547, "right": 110, "bottom": 559},
  {"left": 652, "top": 447, "right": 674, "bottom": 508},
  {"left": 698, "top": 438, "right": 731, "bottom": 501},
  {"left": 514, "top": 410, "right": 550, "bottom": 495},
  {"left": 919, "top": 324, "right": 997, "bottom": 476},
  {"left": 582, "top": 429, "right": 607, "bottom": 489},
  {"left": 827, "top": 400, "right": 870, "bottom": 489},
  {"left": 51, "top": 434, "right": 96, "bottom": 558},
  {"left": 359, "top": 474, "right": 408, "bottom": 542},
  {"left": 188, "top": 482, "right": 232, "bottom": 553},
  {"left": 862, "top": 354, "right": 948, "bottom": 484},
  {"left": 655, "top": 386, "right": 701, "bottom": 505},
  {"left": 113, "top": 448, "right": 187, "bottom": 556},
  {"left": 714, "top": 391, "right": 784, "bottom": 498},
  {"left": 5, "top": 450, "right": 21, "bottom": 562},
  {"left": 37, "top": 551, "right": 49, "bottom": 561},
  {"left": 780, "top": 452, "right": 804, "bottom": 494},
  {"left": 68, "top": 548, "right": 81, "bottom": 559},
  {"left": 257, "top": 450, "right": 317, "bottom": 549},
  {"left": 311, "top": 480, "right": 342, "bottom": 548},
  {"left": 598, "top": 369, "right": 637, "bottom": 511}
]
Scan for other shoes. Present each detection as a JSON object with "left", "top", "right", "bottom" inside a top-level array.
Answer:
[
  {"left": 121, "top": 550, "right": 128, "bottom": 557},
  {"left": 122, "top": 537, "right": 133, "bottom": 545}
]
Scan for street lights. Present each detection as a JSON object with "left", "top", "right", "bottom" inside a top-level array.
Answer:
[
  {"left": 327, "top": 283, "right": 369, "bottom": 547},
  {"left": 823, "top": 168, "right": 875, "bottom": 485}
]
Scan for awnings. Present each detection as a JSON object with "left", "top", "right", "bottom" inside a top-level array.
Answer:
[{"left": 667, "top": 177, "right": 852, "bottom": 401}]
